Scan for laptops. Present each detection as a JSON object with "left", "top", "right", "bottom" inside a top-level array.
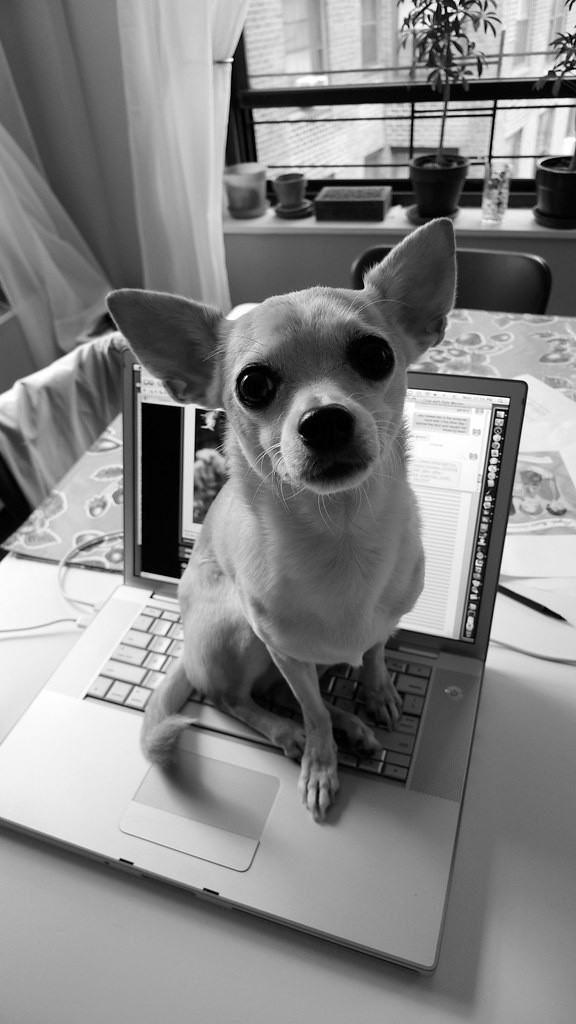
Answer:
[{"left": 1, "top": 344, "right": 525, "bottom": 978}]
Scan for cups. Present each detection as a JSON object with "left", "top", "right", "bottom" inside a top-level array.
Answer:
[
  {"left": 224, "top": 162, "right": 307, "bottom": 214},
  {"left": 482, "top": 163, "right": 509, "bottom": 223}
]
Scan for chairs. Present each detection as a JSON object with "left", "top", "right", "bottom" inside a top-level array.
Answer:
[
  {"left": 355, "top": 243, "right": 553, "bottom": 316},
  {"left": 2, "top": 330, "right": 127, "bottom": 556}
]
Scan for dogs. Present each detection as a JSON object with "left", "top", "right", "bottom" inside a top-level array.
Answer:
[{"left": 102, "top": 216, "right": 460, "bottom": 819}]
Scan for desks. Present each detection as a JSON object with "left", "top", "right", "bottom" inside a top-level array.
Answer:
[{"left": 1, "top": 304, "right": 576, "bottom": 1024}]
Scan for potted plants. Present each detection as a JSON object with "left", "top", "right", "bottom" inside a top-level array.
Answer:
[
  {"left": 397, "top": 0, "right": 501, "bottom": 221},
  {"left": 532, "top": 0, "right": 576, "bottom": 228}
]
